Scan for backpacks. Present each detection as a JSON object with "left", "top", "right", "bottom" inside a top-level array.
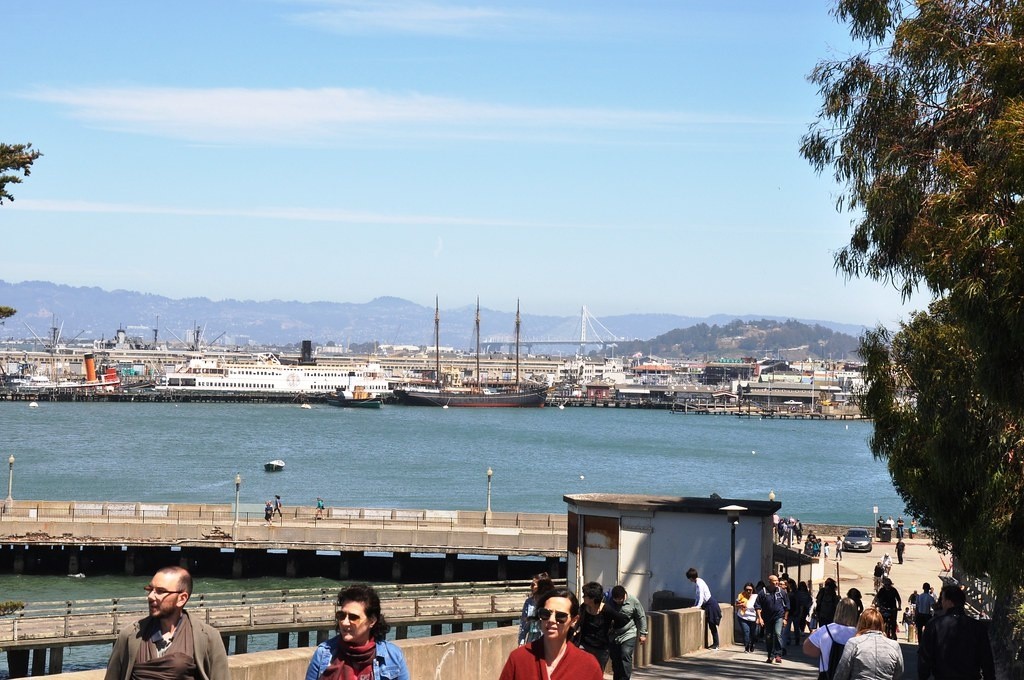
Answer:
[{"left": 795, "top": 522, "right": 800, "bottom": 530}]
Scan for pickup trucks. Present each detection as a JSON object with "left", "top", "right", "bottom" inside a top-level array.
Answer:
[{"left": 783, "top": 400, "right": 803, "bottom": 405}]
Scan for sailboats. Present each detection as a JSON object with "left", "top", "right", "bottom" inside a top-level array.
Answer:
[{"left": 393, "top": 292, "right": 549, "bottom": 408}]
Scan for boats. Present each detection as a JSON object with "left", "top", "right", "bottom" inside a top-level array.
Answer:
[
  {"left": 28, "top": 400, "right": 39, "bottom": 407},
  {"left": 264, "top": 459, "right": 285, "bottom": 471},
  {"left": 155, "top": 349, "right": 395, "bottom": 399},
  {"left": 325, "top": 398, "right": 383, "bottom": 409},
  {"left": 20, "top": 366, "right": 123, "bottom": 392}
]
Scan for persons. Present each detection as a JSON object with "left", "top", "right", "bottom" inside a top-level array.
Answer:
[
  {"left": 778, "top": 518, "right": 843, "bottom": 562},
  {"left": 778, "top": 573, "right": 863, "bottom": 646},
  {"left": 498, "top": 589, "right": 603, "bottom": 680},
  {"left": 754, "top": 575, "right": 791, "bottom": 664},
  {"left": 833, "top": 608, "right": 904, "bottom": 680},
  {"left": 686, "top": 568, "right": 722, "bottom": 650},
  {"left": 305, "top": 585, "right": 410, "bottom": 680},
  {"left": 873, "top": 553, "right": 892, "bottom": 589},
  {"left": 516, "top": 571, "right": 554, "bottom": 648},
  {"left": 902, "top": 582, "right": 937, "bottom": 654},
  {"left": 602, "top": 585, "right": 648, "bottom": 680},
  {"left": 264, "top": 494, "right": 323, "bottom": 522},
  {"left": 803, "top": 599, "right": 858, "bottom": 680},
  {"left": 757, "top": 580, "right": 766, "bottom": 590},
  {"left": 104, "top": 566, "right": 231, "bottom": 680},
  {"left": 878, "top": 516, "right": 917, "bottom": 564},
  {"left": 572, "top": 582, "right": 630, "bottom": 672},
  {"left": 917, "top": 585, "right": 996, "bottom": 680},
  {"left": 736, "top": 582, "right": 759, "bottom": 653},
  {"left": 872, "top": 578, "right": 901, "bottom": 640}
]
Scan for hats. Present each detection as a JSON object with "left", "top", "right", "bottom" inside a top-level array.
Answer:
[
  {"left": 797, "top": 519, "right": 800, "bottom": 521},
  {"left": 809, "top": 530, "right": 813, "bottom": 533}
]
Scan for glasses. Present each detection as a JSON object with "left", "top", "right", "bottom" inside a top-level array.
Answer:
[
  {"left": 885, "top": 583, "right": 891, "bottom": 587},
  {"left": 531, "top": 583, "right": 539, "bottom": 588},
  {"left": 745, "top": 589, "right": 752, "bottom": 591},
  {"left": 538, "top": 608, "right": 571, "bottom": 625},
  {"left": 143, "top": 585, "right": 182, "bottom": 596},
  {"left": 885, "top": 556, "right": 888, "bottom": 557},
  {"left": 334, "top": 610, "right": 370, "bottom": 623},
  {"left": 779, "top": 584, "right": 785, "bottom": 586}
]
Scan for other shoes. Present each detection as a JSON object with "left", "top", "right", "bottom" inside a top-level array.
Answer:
[
  {"left": 776, "top": 656, "right": 782, "bottom": 663},
  {"left": 786, "top": 639, "right": 792, "bottom": 645},
  {"left": 708, "top": 645, "right": 720, "bottom": 650},
  {"left": 744, "top": 649, "right": 749, "bottom": 653},
  {"left": 750, "top": 647, "right": 755, "bottom": 652},
  {"left": 795, "top": 641, "right": 800, "bottom": 646},
  {"left": 766, "top": 657, "right": 774, "bottom": 663}
]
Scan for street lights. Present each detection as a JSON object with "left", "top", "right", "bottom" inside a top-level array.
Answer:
[
  {"left": 1, "top": 454, "right": 15, "bottom": 513},
  {"left": 719, "top": 505, "right": 748, "bottom": 606},
  {"left": 483, "top": 467, "right": 494, "bottom": 527},
  {"left": 231, "top": 470, "right": 242, "bottom": 541}
]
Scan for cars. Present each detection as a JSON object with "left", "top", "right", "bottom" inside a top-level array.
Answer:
[
  {"left": 11, "top": 379, "right": 26, "bottom": 384},
  {"left": 842, "top": 528, "right": 873, "bottom": 552}
]
